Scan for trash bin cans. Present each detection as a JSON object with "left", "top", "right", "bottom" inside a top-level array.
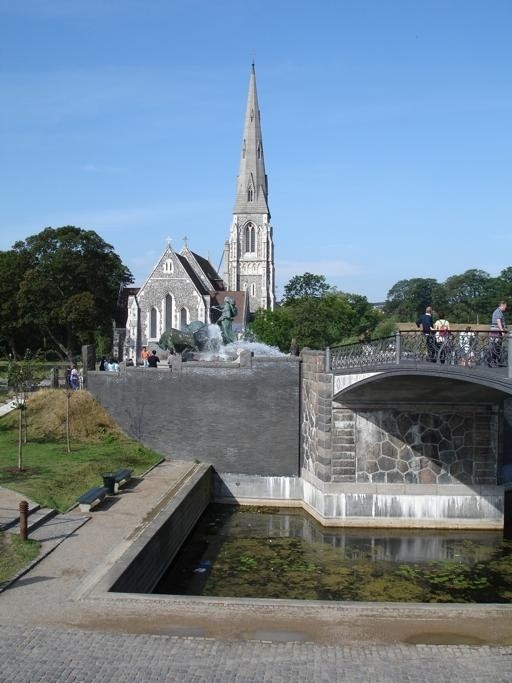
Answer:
[{"left": 103, "top": 477, "right": 115, "bottom": 496}]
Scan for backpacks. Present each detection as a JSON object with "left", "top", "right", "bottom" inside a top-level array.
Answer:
[{"left": 439, "top": 319, "right": 448, "bottom": 337}]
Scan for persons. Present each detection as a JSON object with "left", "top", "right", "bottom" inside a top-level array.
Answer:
[
  {"left": 98, "top": 355, "right": 120, "bottom": 373},
  {"left": 415, "top": 301, "right": 507, "bottom": 369},
  {"left": 139, "top": 347, "right": 174, "bottom": 368},
  {"left": 212, "top": 297, "right": 238, "bottom": 341},
  {"left": 290, "top": 338, "right": 301, "bottom": 355},
  {"left": 64, "top": 365, "right": 84, "bottom": 390}
]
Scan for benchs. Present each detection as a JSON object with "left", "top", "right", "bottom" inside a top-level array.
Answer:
[
  {"left": 77, "top": 487, "right": 109, "bottom": 511},
  {"left": 101, "top": 467, "right": 137, "bottom": 493}
]
